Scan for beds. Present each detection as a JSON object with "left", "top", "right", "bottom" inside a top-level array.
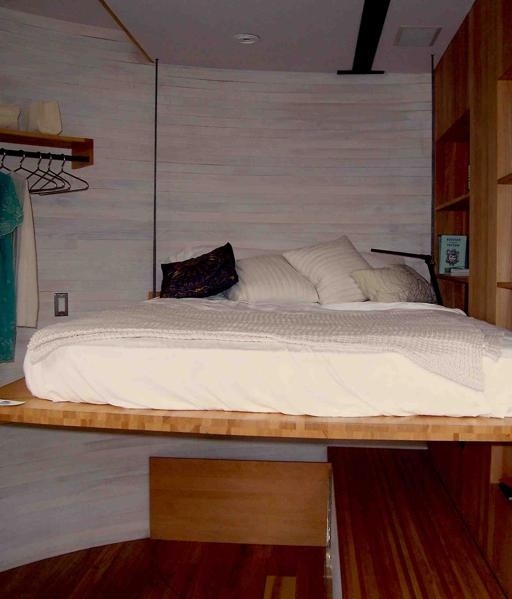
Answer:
[{"left": 0, "top": 244, "right": 512, "bottom": 442}]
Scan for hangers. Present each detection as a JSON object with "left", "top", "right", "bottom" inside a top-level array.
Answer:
[{"left": 0, "top": 148, "right": 89, "bottom": 196}]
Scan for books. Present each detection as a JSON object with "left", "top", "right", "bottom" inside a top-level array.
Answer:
[{"left": 438, "top": 235, "right": 469, "bottom": 275}]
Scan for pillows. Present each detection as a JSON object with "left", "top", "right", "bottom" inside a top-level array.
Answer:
[{"left": 159, "top": 234, "right": 438, "bottom": 305}]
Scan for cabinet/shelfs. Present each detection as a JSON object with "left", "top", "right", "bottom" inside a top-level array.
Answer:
[{"left": 432, "top": 0, "right": 512, "bottom": 331}]
